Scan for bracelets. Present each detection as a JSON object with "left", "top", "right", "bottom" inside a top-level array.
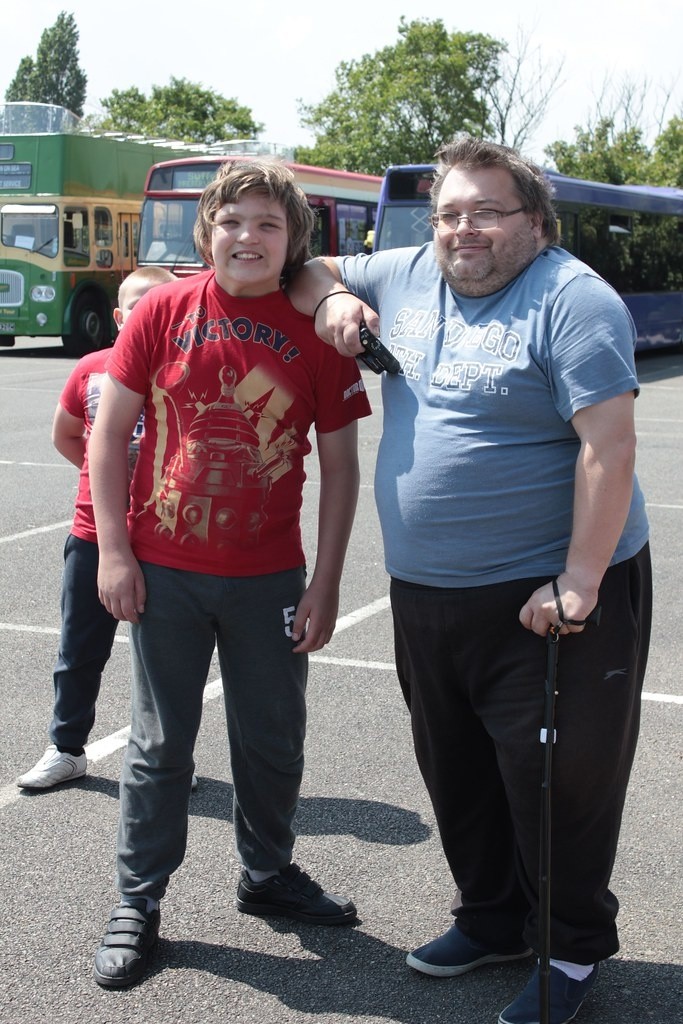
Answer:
[{"left": 314, "top": 290, "right": 359, "bottom": 322}]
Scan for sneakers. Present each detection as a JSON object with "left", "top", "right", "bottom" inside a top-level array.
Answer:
[{"left": 14, "top": 743, "right": 89, "bottom": 789}]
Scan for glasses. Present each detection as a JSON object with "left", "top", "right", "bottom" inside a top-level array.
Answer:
[{"left": 427, "top": 206, "right": 527, "bottom": 233}]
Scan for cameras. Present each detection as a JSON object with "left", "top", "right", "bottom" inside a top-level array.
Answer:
[{"left": 356, "top": 322, "right": 401, "bottom": 375}]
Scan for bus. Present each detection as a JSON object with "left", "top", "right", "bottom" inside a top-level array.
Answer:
[
  {"left": 361, "top": 163, "right": 682, "bottom": 354},
  {"left": 0, "top": 132, "right": 229, "bottom": 358},
  {"left": 136, "top": 155, "right": 384, "bottom": 278}
]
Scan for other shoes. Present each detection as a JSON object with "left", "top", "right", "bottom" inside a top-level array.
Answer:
[
  {"left": 407, "top": 919, "right": 534, "bottom": 974},
  {"left": 235, "top": 864, "right": 357, "bottom": 926},
  {"left": 91, "top": 898, "right": 160, "bottom": 985},
  {"left": 499, "top": 956, "right": 601, "bottom": 1023}
]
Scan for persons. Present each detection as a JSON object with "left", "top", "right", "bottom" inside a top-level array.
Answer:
[
  {"left": 87, "top": 156, "right": 373, "bottom": 991},
  {"left": 15, "top": 265, "right": 199, "bottom": 793},
  {"left": 287, "top": 143, "right": 653, "bottom": 1023}
]
